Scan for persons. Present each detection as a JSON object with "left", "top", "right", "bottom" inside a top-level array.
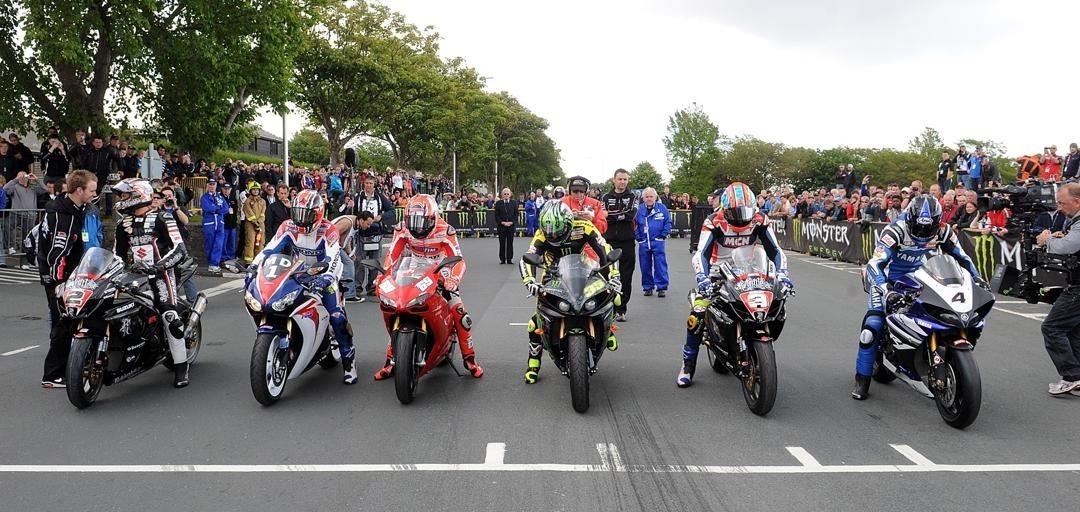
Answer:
[
  {"left": 1036, "top": 182, "right": 1080, "bottom": 400},
  {"left": 494, "top": 187, "right": 518, "bottom": 265},
  {"left": 347, "top": 178, "right": 396, "bottom": 297},
  {"left": 520, "top": 185, "right": 566, "bottom": 236},
  {"left": 557, "top": 177, "right": 609, "bottom": 236},
  {"left": 662, "top": 141, "right": 1080, "bottom": 232},
  {"left": 330, "top": 212, "right": 373, "bottom": 303},
  {"left": 1, "top": 123, "right": 492, "bottom": 274},
  {"left": 677, "top": 182, "right": 795, "bottom": 388},
  {"left": 373, "top": 195, "right": 484, "bottom": 381},
  {"left": 851, "top": 194, "right": 989, "bottom": 400},
  {"left": 41, "top": 170, "right": 99, "bottom": 389},
  {"left": 112, "top": 178, "right": 190, "bottom": 388},
  {"left": 519, "top": 199, "right": 618, "bottom": 384},
  {"left": 602, "top": 168, "right": 639, "bottom": 322},
  {"left": 162, "top": 188, "right": 197, "bottom": 303},
  {"left": 633, "top": 187, "right": 671, "bottom": 297}
]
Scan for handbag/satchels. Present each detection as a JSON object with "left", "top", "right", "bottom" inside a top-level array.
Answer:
[{"left": 357, "top": 219, "right": 386, "bottom": 237}]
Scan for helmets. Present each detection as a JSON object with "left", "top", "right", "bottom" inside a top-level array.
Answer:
[
  {"left": 904, "top": 192, "right": 944, "bottom": 249},
  {"left": 536, "top": 199, "right": 574, "bottom": 248},
  {"left": 720, "top": 179, "right": 758, "bottom": 232},
  {"left": 402, "top": 193, "right": 442, "bottom": 243},
  {"left": 109, "top": 176, "right": 154, "bottom": 214},
  {"left": 290, "top": 188, "right": 325, "bottom": 235},
  {"left": 552, "top": 185, "right": 566, "bottom": 198},
  {"left": 246, "top": 180, "right": 263, "bottom": 191}
]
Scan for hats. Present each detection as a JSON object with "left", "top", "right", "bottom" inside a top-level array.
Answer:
[
  {"left": 205, "top": 178, "right": 218, "bottom": 183},
  {"left": 707, "top": 186, "right": 724, "bottom": 197},
  {"left": 900, "top": 186, "right": 911, "bottom": 194},
  {"left": 221, "top": 182, "right": 233, "bottom": 188},
  {"left": 570, "top": 177, "right": 588, "bottom": 193}
]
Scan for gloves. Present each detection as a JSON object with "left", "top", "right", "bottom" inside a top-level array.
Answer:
[
  {"left": 975, "top": 280, "right": 992, "bottom": 296},
  {"left": 129, "top": 262, "right": 147, "bottom": 275},
  {"left": 443, "top": 274, "right": 460, "bottom": 293},
  {"left": 148, "top": 264, "right": 164, "bottom": 275},
  {"left": 883, "top": 290, "right": 909, "bottom": 312},
  {"left": 372, "top": 273, "right": 384, "bottom": 288},
  {"left": 604, "top": 277, "right": 626, "bottom": 305},
  {"left": 523, "top": 280, "right": 544, "bottom": 299},
  {"left": 697, "top": 276, "right": 715, "bottom": 298},
  {"left": 774, "top": 273, "right": 796, "bottom": 297},
  {"left": 301, "top": 274, "right": 335, "bottom": 295},
  {"left": 244, "top": 268, "right": 256, "bottom": 289}
]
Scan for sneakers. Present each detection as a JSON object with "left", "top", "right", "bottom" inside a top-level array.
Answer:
[
  {"left": 41, "top": 373, "right": 67, "bottom": 388},
  {"left": 342, "top": 355, "right": 358, "bottom": 385},
  {"left": 643, "top": 288, "right": 654, "bottom": 298},
  {"left": 606, "top": 328, "right": 619, "bottom": 352},
  {"left": 655, "top": 288, "right": 667, "bottom": 299},
  {"left": 1046, "top": 378, "right": 1080, "bottom": 397},
  {"left": 373, "top": 359, "right": 395, "bottom": 381},
  {"left": 849, "top": 373, "right": 872, "bottom": 402},
  {"left": 614, "top": 312, "right": 628, "bottom": 324},
  {"left": 345, "top": 296, "right": 366, "bottom": 304},
  {"left": 462, "top": 357, "right": 485, "bottom": 379},
  {"left": 524, "top": 357, "right": 541, "bottom": 385},
  {"left": 673, "top": 356, "right": 696, "bottom": 390},
  {"left": 207, "top": 263, "right": 224, "bottom": 274},
  {"left": 173, "top": 362, "right": 190, "bottom": 388}
]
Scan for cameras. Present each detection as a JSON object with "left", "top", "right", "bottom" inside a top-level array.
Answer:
[
  {"left": 23, "top": 173, "right": 34, "bottom": 181},
  {"left": 164, "top": 197, "right": 173, "bottom": 206}
]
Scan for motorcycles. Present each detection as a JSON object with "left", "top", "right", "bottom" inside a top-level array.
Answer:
[
  {"left": 859, "top": 253, "right": 1000, "bottom": 432},
  {"left": 523, "top": 247, "right": 626, "bottom": 414},
  {"left": 688, "top": 245, "right": 795, "bottom": 417},
  {"left": 57, "top": 244, "right": 208, "bottom": 409},
  {"left": 360, "top": 255, "right": 465, "bottom": 404},
  {"left": 233, "top": 258, "right": 351, "bottom": 405}
]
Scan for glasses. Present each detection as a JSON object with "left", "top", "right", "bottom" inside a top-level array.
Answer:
[{"left": 901, "top": 192, "right": 909, "bottom": 194}]
